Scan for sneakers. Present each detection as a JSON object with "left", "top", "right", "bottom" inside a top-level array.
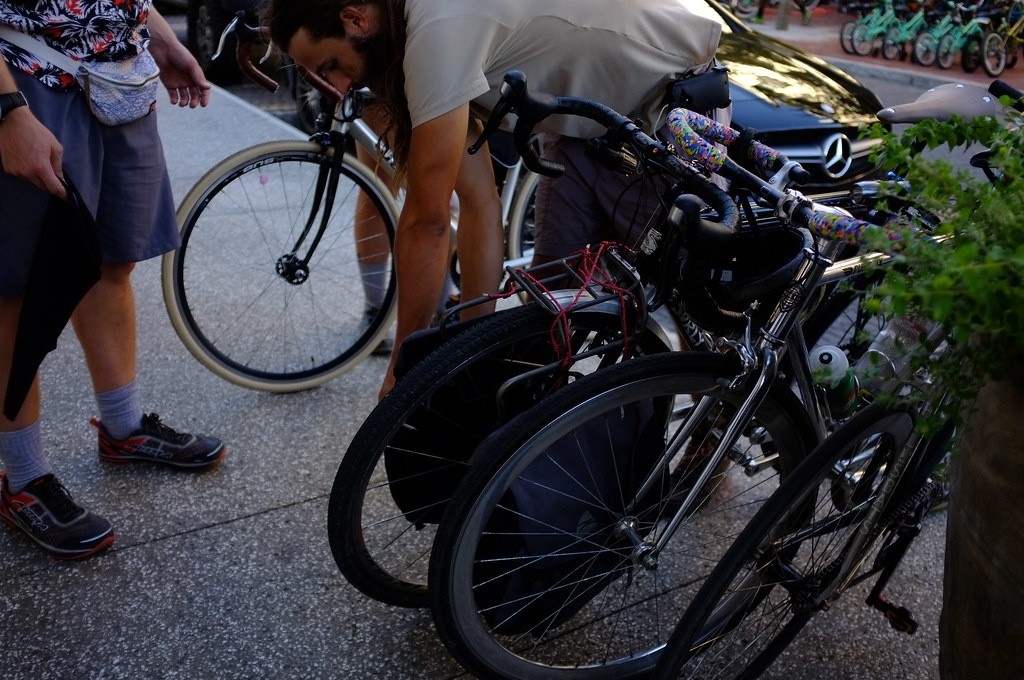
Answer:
[
  {"left": 0, "top": 469, "right": 116, "bottom": 561},
  {"left": 88, "top": 412, "right": 227, "bottom": 473}
]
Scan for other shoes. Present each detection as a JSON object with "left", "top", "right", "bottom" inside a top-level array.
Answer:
[{"left": 359, "top": 306, "right": 395, "bottom": 356}]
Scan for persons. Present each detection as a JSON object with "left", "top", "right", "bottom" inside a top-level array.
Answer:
[
  {"left": 330, "top": 71, "right": 457, "bottom": 356},
  {"left": 269, "top": 0, "right": 732, "bottom": 405},
  {"left": 0, "top": 0, "right": 226, "bottom": 561}
]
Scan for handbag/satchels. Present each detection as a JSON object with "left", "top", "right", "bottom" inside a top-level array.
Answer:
[
  {"left": 72, "top": 47, "right": 162, "bottom": 127},
  {"left": 375, "top": 312, "right": 568, "bottom": 534},
  {"left": 449, "top": 388, "right": 670, "bottom": 631}
]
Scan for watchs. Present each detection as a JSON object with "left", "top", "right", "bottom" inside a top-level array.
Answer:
[{"left": 0, "top": 91, "right": 30, "bottom": 122}]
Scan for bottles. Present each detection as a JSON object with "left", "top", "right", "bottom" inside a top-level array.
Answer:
[
  {"left": 809, "top": 346, "right": 862, "bottom": 420},
  {"left": 856, "top": 315, "right": 931, "bottom": 399}
]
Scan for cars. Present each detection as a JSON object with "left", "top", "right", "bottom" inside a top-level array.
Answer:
[{"left": 186, "top": 0, "right": 913, "bottom": 264}]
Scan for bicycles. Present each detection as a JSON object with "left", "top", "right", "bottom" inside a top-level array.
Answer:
[{"left": 157, "top": 3, "right": 1024, "bottom": 678}]
